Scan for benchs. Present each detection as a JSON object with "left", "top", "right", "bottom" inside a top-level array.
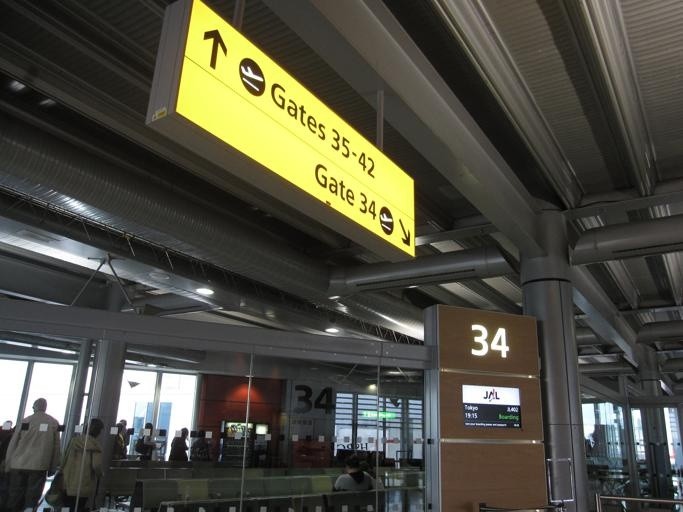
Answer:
[{"left": 101, "top": 459, "right": 425, "bottom": 512}]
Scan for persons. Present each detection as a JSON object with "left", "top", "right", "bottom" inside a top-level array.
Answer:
[
  {"left": 111, "top": 420, "right": 213, "bottom": 463},
  {"left": 331, "top": 455, "right": 376, "bottom": 493},
  {"left": 0, "top": 396, "right": 106, "bottom": 511}
]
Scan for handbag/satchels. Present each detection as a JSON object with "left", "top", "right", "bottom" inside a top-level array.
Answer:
[
  {"left": 45, "top": 472, "right": 65, "bottom": 507},
  {"left": 135, "top": 439, "right": 148, "bottom": 453}
]
